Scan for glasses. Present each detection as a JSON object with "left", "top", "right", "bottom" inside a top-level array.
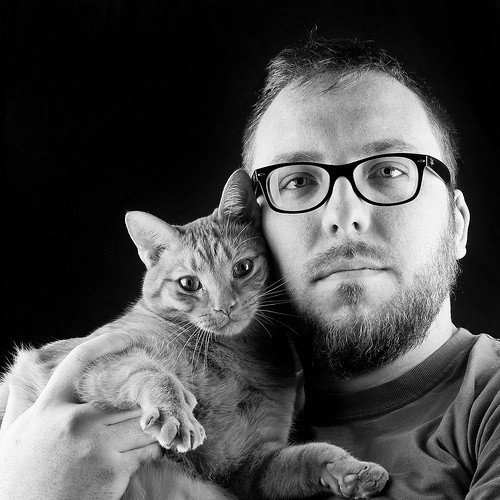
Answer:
[{"left": 252, "top": 153, "right": 450, "bottom": 214}]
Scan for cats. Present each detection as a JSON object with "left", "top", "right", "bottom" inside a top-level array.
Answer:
[{"left": 0, "top": 167, "right": 391, "bottom": 500}]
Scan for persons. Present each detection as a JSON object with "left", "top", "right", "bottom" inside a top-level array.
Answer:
[{"left": 0, "top": 40, "right": 500, "bottom": 500}]
